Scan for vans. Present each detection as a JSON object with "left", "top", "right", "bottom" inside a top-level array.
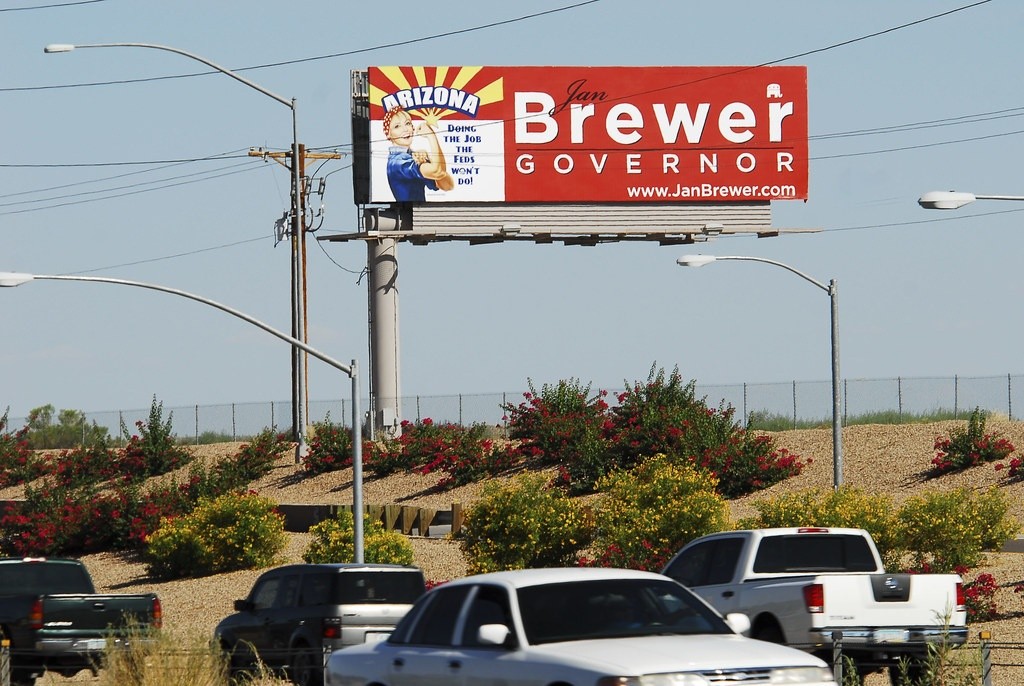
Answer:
[{"left": 208, "top": 562, "right": 434, "bottom": 685}]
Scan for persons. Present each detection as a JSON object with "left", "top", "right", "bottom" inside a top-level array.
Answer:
[{"left": 382, "top": 106, "right": 454, "bottom": 201}]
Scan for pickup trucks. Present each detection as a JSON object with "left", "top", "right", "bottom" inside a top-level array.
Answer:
[
  {"left": 654, "top": 525, "right": 971, "bottom": 686},
  {"left": 0, "top": 557, "right": 162, "bottom": 686}
]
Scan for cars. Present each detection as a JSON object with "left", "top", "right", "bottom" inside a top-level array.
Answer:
[{"left": 321, "top": 567, "right": 838, "bottom": 686}]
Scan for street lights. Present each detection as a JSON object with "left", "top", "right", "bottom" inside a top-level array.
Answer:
[
  {"left": 676, "top": 255, "right": 842, "bottom": 495},
  {"left": 1, "top": 270, "right": 365, "bottom": 567},
  {"left": 44, "top": 43, "right": 310, "bottom": 464}
]
